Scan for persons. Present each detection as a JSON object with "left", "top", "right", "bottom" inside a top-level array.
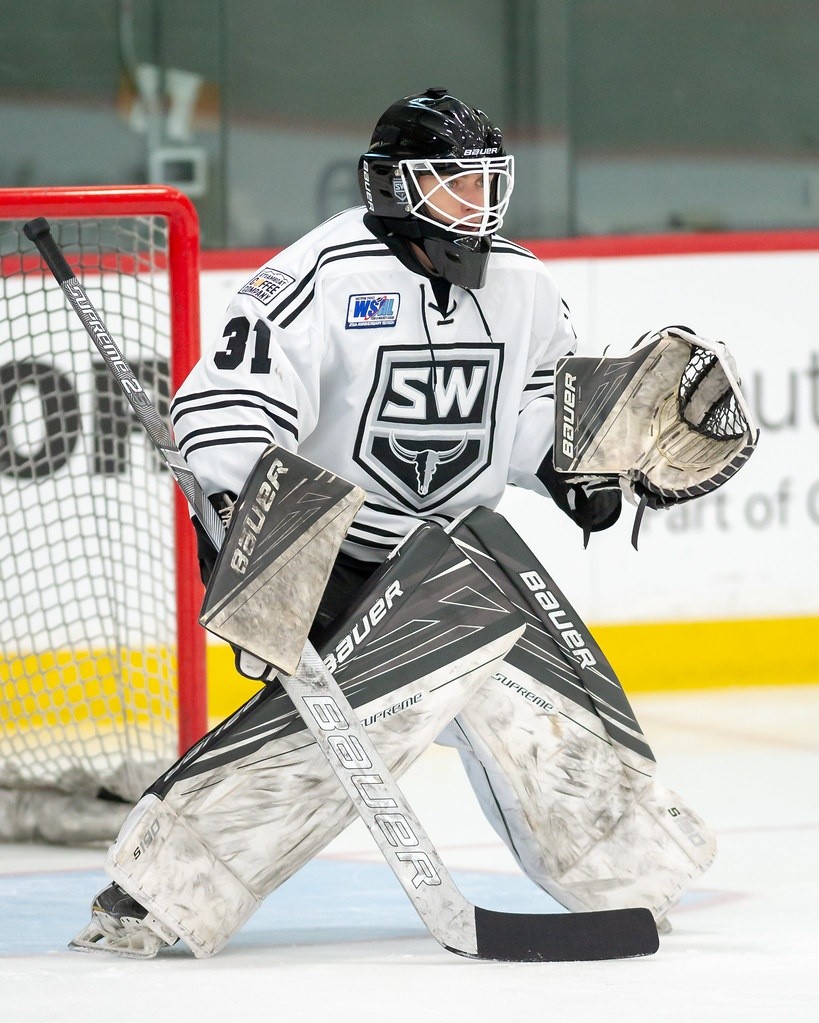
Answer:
[{"left": 64, "top": 86, "right": 760, "bottom": 959}]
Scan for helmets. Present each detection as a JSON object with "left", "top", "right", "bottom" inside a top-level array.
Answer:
[{"left": 358, "top": 87, "right": 514, "bottom": 289}]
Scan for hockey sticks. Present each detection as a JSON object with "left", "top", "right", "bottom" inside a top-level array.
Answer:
[{"left": 22, "top": 215, "right": 661, "bottom": 965}]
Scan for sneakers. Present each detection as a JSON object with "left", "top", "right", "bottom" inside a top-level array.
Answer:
[{"left": 67, "top": 879, "right": 181, "bottom": 960}]
